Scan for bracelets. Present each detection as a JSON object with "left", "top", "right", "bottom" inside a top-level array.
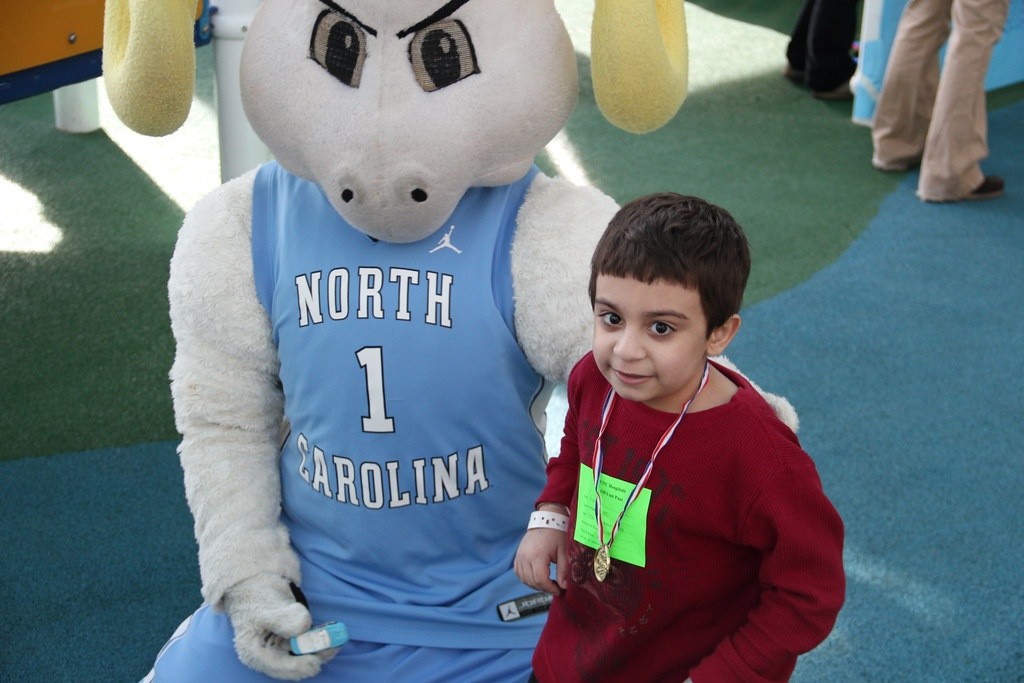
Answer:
[{"left": 528, "top": 511, "right": 570, "bottom": 532}]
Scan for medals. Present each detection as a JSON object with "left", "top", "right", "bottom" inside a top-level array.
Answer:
[{"left": 593, "top": 545, "right": 610, "bottom": 582}]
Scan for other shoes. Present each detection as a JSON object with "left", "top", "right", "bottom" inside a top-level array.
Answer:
[
  {"left": 786, "top": 64, "right": 805, "bottom": 81},
  {"left": 871, "top": 152, "right": 922, "bottom": 172},
  {"left": 811, "top": 78, "right": 853, "bottom": 100},
  {"left": 960, "top": 174, "right": 1004, "bottom": 198}
]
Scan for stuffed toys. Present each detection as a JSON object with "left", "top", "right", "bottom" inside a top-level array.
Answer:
[{"left": 102, "top": 0, "right": 691, "bottom": 683}]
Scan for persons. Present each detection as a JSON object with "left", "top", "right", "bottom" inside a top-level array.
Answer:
[
  {"left": 785, "top": 0, "right": 858, "bottom": 103},
  {"left": 515, "top": 192, "right": 846, "bottom": 683},
  {"left": 871, "top": 0, "right": 1010, "bottom": 203}
]
